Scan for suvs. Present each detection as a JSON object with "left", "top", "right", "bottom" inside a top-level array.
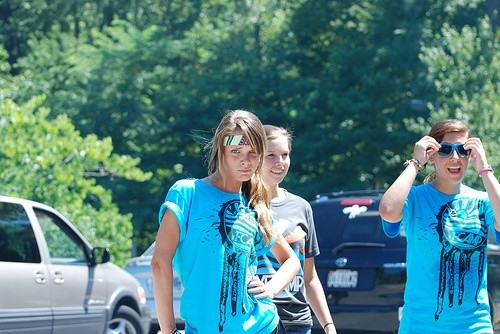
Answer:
[{"left": 307, "top": 191, "right": 500, "bottom": 334}]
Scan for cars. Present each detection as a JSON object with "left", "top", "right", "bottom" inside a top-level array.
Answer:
[{"left": 124, "top": 240, "right": 185, "bottom": 334}]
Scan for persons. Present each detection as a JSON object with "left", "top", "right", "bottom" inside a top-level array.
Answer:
[
  {"left": 152, "top": 111, "right": 301, "bottom": 334},
  {"left": 243, "top": 123, "right": 336, "bottom": 334},
  {"left": 378, "top": 117, "right": 499, "bottom": 333}
]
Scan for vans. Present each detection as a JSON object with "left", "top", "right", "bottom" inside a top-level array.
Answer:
[{"left": 0, "top": 195, "right": 151, "bottom": 334}]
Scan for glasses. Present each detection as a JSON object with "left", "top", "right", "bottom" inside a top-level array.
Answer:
[{"left": 436, "top": 143, "right": 472, "bottom": 158}]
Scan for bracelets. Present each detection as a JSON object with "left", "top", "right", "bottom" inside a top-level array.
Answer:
[
  {"left": 320, "top": 320, "right": 333, "bottom": 329},
  {"left": 478, "top": 167, "right": 495, "bottom": 176},
  {"left": 171, "top": 328, "right": 181, "bottom": 334},
  {"left": 403, "top": 158, "right": 423, "bottom": 171}
]
